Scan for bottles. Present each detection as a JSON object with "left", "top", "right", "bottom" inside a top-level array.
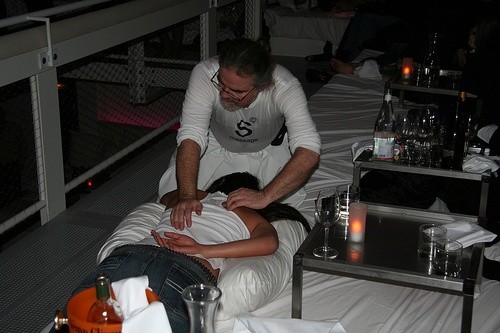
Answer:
[
  {"left": 372, "top": 88, "right": 395, "bottom": 160},
  {"left": 183, "top": 283, "right": 223, "bottom": 333},
  {"left": 426, "top": 33, "right": 441, "bottom": 69},
  {"left": 443, "top": 97, "right": 465, "bottom": 170},
  {"left": 87, "top": 276, "right": 121, "bottom": 324},
  {"left": 459, "top": 92, "right": 469, "bottom": 143}
]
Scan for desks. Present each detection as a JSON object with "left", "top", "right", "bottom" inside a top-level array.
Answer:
[
  {"left": 353, "top": 147, "right": 491, "bottom": 229},
  {"left": 383, "top": 70, "right": 482, "bottom": 121},
  {"left": 292, "top": 200, "right": 487, "bottom": 333}
]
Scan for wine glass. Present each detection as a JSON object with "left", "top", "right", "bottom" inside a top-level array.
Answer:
[
  {"left": 396, "top": 112, "right": 415, "bottom": 163},
  {"left": 314, "top": 189, "right": 340, "bottom": 258},
  {"left": 415, "top": 116, "right": 432, "bottom": 161}
]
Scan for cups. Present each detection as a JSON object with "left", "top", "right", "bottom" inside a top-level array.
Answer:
[
  {"left": 418, "top": 224, "right": 447, "bottom": 260},
  {"left": 336, "top": 184, "right": 361, "bottom": 219},
  {"left": 433, "top": 238, "right": 462, "bottom": 275}
]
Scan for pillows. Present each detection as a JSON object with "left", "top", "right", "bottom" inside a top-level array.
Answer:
[
  {"left": 216, "top": 210, "right": 317, "bottom": 321},
  {"left": 97, "top": 202, "right": 166, "bottom": 261}
]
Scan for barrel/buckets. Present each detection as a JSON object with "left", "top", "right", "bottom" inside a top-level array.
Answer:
[{"left": 66, "top": 283, "right": 158, "bottom": 333}]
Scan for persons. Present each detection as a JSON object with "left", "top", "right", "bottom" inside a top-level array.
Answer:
[
  {"left": 49, "top": 172, "right": 311, "bottom": 333},
  {"left": 278, "top": 0, "right": 427, "bottom": 75},
  {"left": 154, "top": 36, "right": 321, "bottom": 230}
]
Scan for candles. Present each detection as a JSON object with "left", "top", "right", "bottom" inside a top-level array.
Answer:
[{"left": 349, "top": 198, "right": 367, "bottom": 242}]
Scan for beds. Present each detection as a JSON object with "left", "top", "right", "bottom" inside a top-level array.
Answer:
[
  {"left": 250, "top": 49, "right": 499, "bottom": 333},
  {"left": 263, "top": 0, "right": 353, "bottom": 57}
]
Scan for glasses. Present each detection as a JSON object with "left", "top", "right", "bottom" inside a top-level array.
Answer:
[{"left": 210, "top": 68, "right": 255, "bottom": 102}]
